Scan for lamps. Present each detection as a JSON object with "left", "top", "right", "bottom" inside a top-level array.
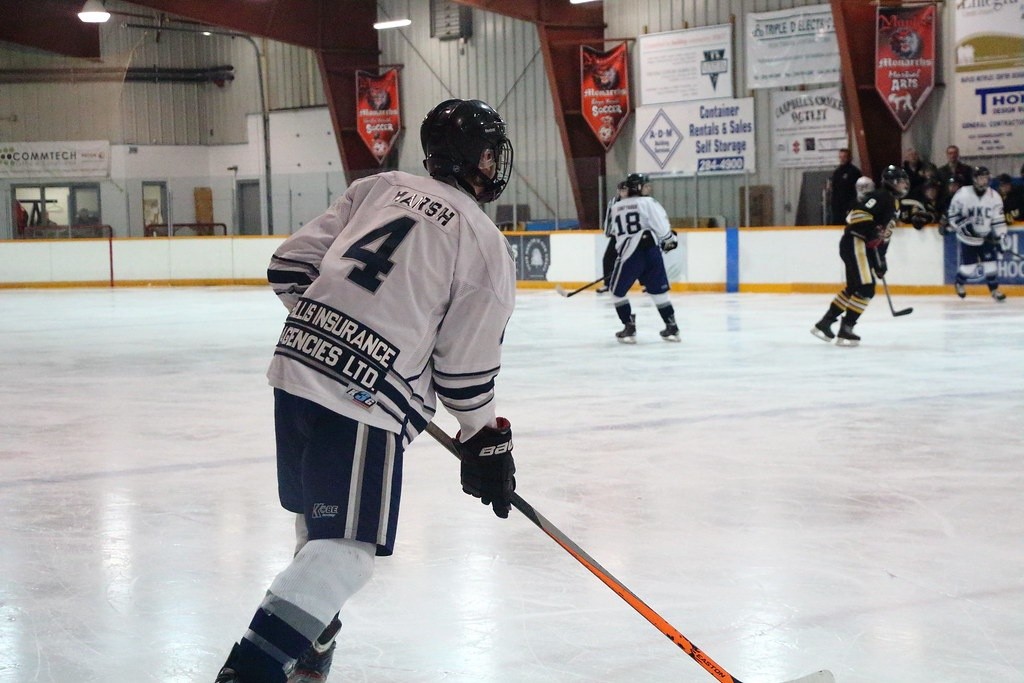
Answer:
[{"left": 78, "top": 0, "right": 111, "bottom": 23}]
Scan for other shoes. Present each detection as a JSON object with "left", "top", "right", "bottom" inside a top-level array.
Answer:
[{"left": 595, "top": 285, "right": 610, "bottom": 296}]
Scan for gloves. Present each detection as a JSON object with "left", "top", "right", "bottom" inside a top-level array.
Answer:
[
  {"left": 910, "top": 212, "right": 934, "bottom": 229},
  {"left": 872, "top": 255, "right": 887, "bottom": 278},
  {"left": 661, "top": 230, "right": 678, "bottom": 250},
  {"left": 995, "top": 234, "right": 1008, "bottom": 253},
  {"left": 956, "top": 220, "right": 977, "bottom": 238},
  {"left": 863, "top": 227, "right": 881, "bottom": 248},
  {"left": 452, "top": 417, "right": 516, "bottom": 518}
]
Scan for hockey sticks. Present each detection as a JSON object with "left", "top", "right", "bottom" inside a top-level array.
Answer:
[
  {"left": 556, "top": 277, "right": 604, "bottom": 298},
  {"left": 994, "top": 244, "right": 1024, "bottom": 260},
  {"left": 875, "top": 247, "right": 913, "bottom": 317},
  {"left": 424, "top": 419, "right": 837, "bottom": 683}
]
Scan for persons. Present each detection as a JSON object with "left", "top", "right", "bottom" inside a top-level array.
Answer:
[
  {"left": 947, "top": 167, "right": 1013, "bottom": 303},
  {"left": 33, "top": 207, "right": 100, "bottom": 226},
  {"left": 808, "top": 163, "right": 910, "bottom": 347},
  {"left": 211, "top": 98, "right": 519, "bottom": 683},
  {"left": 596, "top": 172, "right": 682, "bottom": 343},
  {"left": 826, "top": 145, "right": 1024, "bottom": 231}
]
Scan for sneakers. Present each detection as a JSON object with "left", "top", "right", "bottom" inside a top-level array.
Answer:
[
  {"left": 214, "top": 639, "right": 288, "bottom": 683},
  {"left": 286, "top": 618, "right": 341, "bottom": 682},
  {"left": 835, "top": 317, "right": 860, "bottom": 347},
  {"left": 659, "top": 316, "right": 681, "bottom": 342},
  {"left": 616, "top": 315, "right": 637, "bottom": 344},
  {"left": 810, "top": 312, "right": 838, "bottom": 342},
  {"left": 954, "top": 281, "right": 967, "bottom": 300},
  {"left": 990, "top": 288, "right": 1006, "bottom": 302}
]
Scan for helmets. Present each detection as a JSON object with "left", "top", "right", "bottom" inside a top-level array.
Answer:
[
  {"left": 924, "top": 179, "right": 941, "bottom": 193},
  {"left": 625, "top": 172, "right": 650, "bottom": 192},
  {"left": 617, "top": 181, "right": 628, "bottom": 188},
  {"left": 421, "top": 99, "right": 513, "bottom": 202},
  {"left": 972, "top": 166, "right": 990, "bottom": 180},
  {"left": 945, "top": 175, "right": 961, "bottom": 187},
  {"left": 883, "top": 165, "right": 908, "bottom": 184}
]
